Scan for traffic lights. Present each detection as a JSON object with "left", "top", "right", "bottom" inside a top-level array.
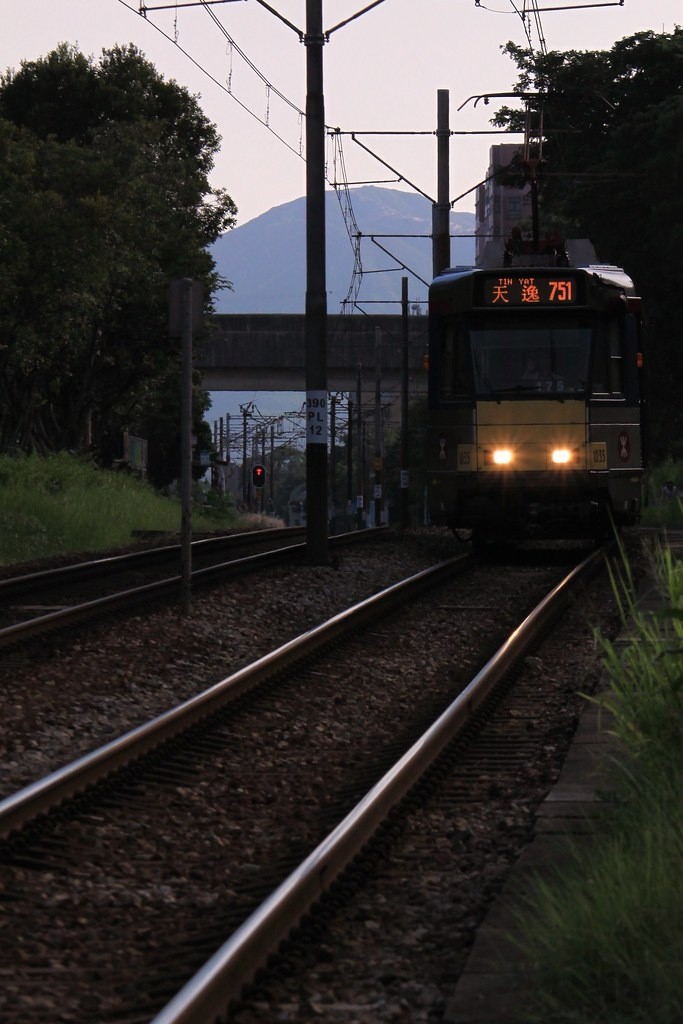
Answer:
[{"left": 253, "top": 465, "right": 266, "bottom": 486}]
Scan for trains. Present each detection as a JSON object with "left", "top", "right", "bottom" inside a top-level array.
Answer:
[{"left": 424, "top": 258, "right": 646, "bottom": 552}]
